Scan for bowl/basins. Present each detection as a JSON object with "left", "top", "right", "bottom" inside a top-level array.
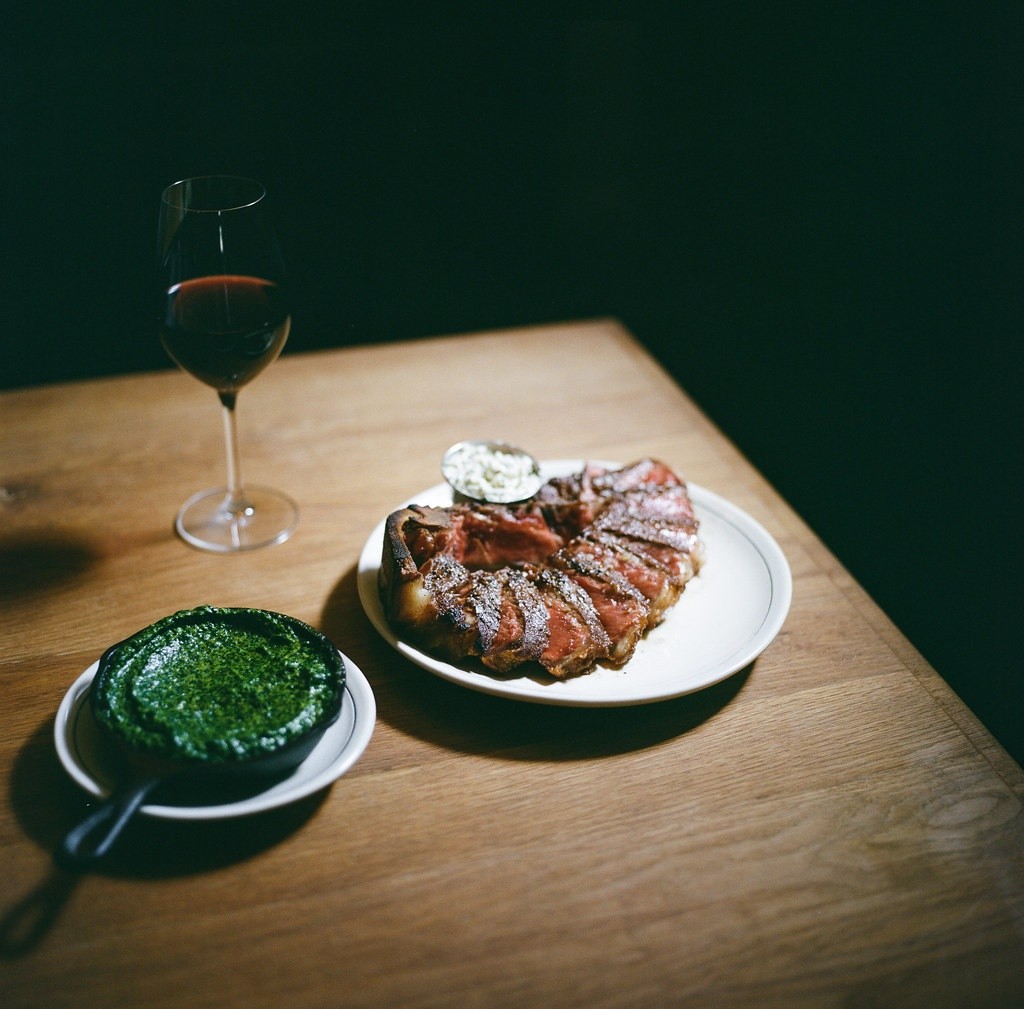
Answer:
[
  {"left": 441, "top": 438, "right": 544, "bottom": 507},
  {"left": 90, "top": 607, "right": 347, "bottom": 804}
]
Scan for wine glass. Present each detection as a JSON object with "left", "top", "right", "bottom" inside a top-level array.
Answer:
[{"left": 153, "top": 175, "right": 301, "bottom": 553}]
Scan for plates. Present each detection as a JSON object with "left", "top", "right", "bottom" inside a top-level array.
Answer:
[
  {"left": 53, "top": 647, "right": 377, "bottom": 819},
  {"left": 357, "top": 459, "right": 792, "bottom": 708}
]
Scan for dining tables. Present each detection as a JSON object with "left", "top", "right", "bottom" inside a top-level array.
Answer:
[{"left": 1, "top": 314, "right": 1024, "bottom": 1008}]
